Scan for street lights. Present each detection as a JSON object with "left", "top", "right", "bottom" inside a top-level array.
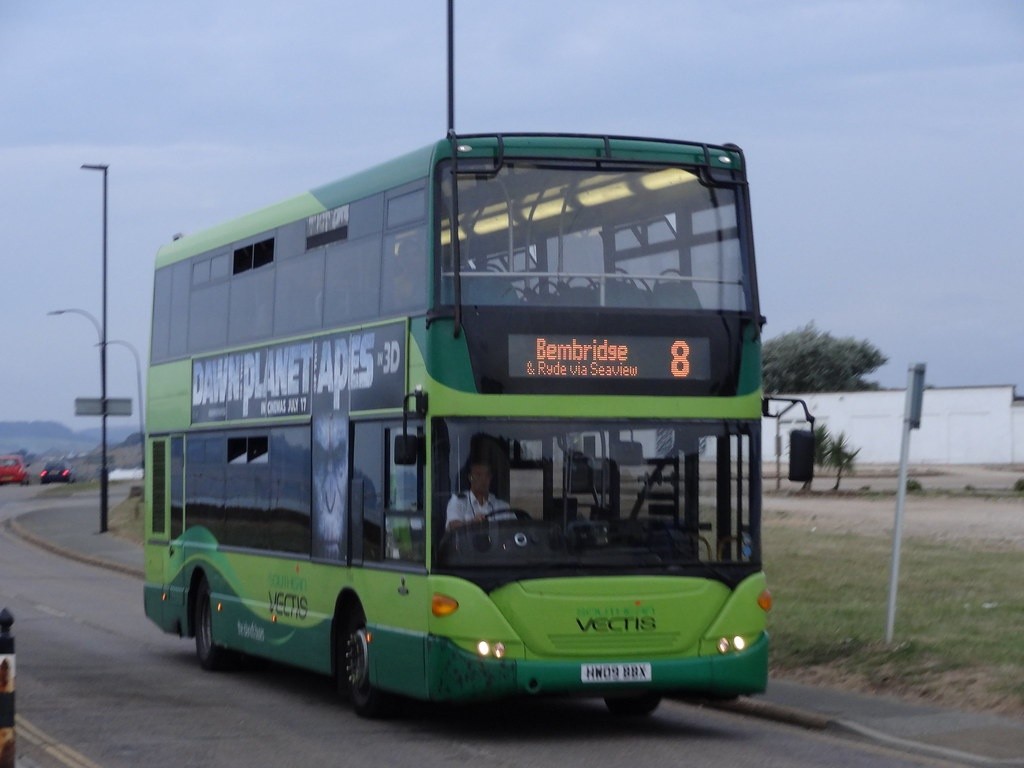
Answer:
[
  {"left": 95, "top": 339, "right": 145, "bottom": 485},
  {"left": 47, "top": 164, "right": 110, "bottom": 533}
]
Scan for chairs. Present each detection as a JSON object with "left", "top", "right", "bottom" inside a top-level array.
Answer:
[{"left": 466, "top": 263, "right": 704, "bottom": 309}]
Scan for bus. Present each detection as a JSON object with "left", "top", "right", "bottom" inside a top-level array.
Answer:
[{"left": 145, "top": 131, "right": 820, "bottom": 720}]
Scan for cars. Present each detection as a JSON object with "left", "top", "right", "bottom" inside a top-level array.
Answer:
[{"left": 39, "top": 461, "right": 78, "bottom": 483}]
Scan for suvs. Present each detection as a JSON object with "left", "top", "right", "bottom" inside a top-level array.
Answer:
[{"left": 0, "top": 455, "right": 33, "bottom": 486}]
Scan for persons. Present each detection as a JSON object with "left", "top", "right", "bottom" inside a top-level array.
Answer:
[{"left": 443, "top": 459, "right": 518, "bottom": 534}]
